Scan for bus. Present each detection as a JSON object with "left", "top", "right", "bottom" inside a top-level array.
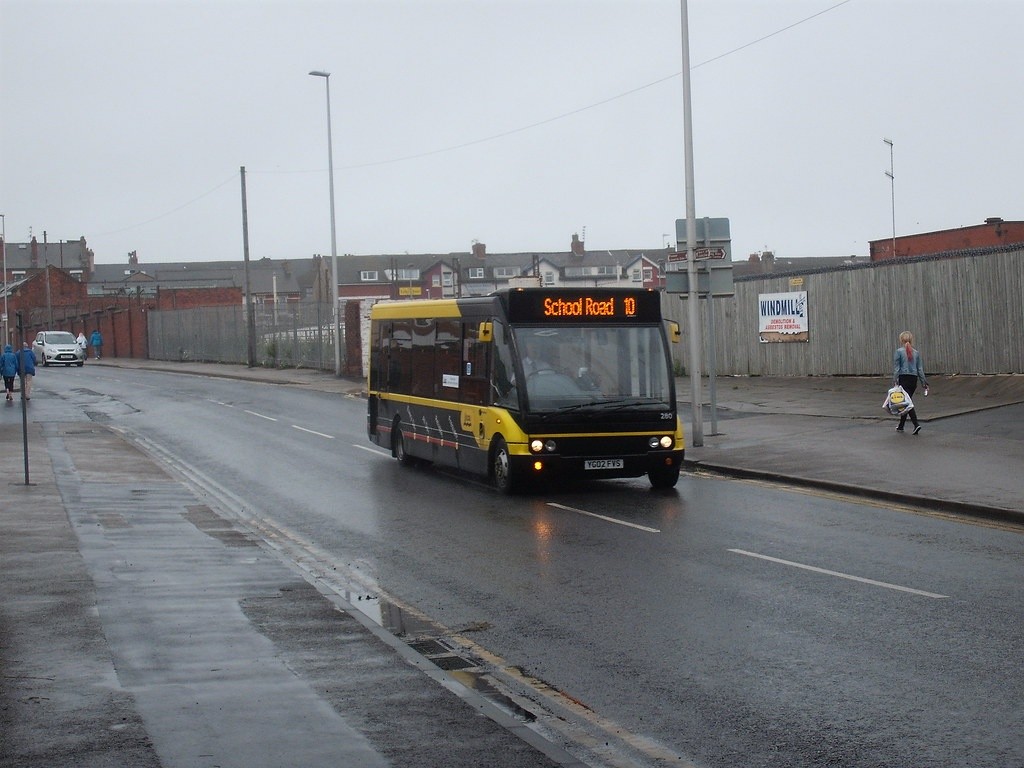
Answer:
[{"left": 367, "top": 287, "right": 686, "bottom": 488}]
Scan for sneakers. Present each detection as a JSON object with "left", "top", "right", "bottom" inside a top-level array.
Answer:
[
  {"left": 912, "top": 426, "right": 921, "bottom": 435},
  {"left": 895, "top": 427, "right": 904, "bottom": 431}
]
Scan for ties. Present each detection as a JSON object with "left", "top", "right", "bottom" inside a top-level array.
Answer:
[{"left": 531, "top": 362, "right": 538, "bottom": 373}]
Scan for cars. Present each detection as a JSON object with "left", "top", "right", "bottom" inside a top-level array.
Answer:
[{"left": 31, "top": 331, "right": 85, "bottom": 367}]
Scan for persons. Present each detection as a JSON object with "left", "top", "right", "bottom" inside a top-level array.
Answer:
[
  {"left": 894, "top": 331, "right": 929, "bottom": 435},
  {"left": 89, "top": 329, "right": 104, "bottom": 360},
  {"left": 76, "top": 333, "right": 88, "bottom": 361},
  {"left": 511, "top": 335, "right": 550, "bottom": 385},
  {"left": 0, "top": 344, "right": 18, "bottom": 400},
  {"left": 15, "top": 342, "right": 38, "bottom": 400}
]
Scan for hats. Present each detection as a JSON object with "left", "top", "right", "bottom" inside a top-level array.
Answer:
[{"left": 23, "top": 342, "right": 28, "bottom": 346}]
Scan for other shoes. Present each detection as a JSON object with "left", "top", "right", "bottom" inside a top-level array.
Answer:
[
  {"left": 95, "top": 356, "right": 101, "bottom": 360},
  {"left": 6, "top": 394, "right": 13, "bottom": 400},
  {"left": 21, "top": 397, "right": 30, "bottom": 400}
]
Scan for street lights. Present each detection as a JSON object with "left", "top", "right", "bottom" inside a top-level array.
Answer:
[
  {"left": 0, "top": 214, "right": 8, "bottom": 345},
  {"left": 309, "top": 71, "right": 342, "bottom": 377}
]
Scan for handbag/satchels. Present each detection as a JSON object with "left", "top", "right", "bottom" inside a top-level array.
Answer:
[{"left": 882, "top": 381, "right": 915, "bottom": 416}]
